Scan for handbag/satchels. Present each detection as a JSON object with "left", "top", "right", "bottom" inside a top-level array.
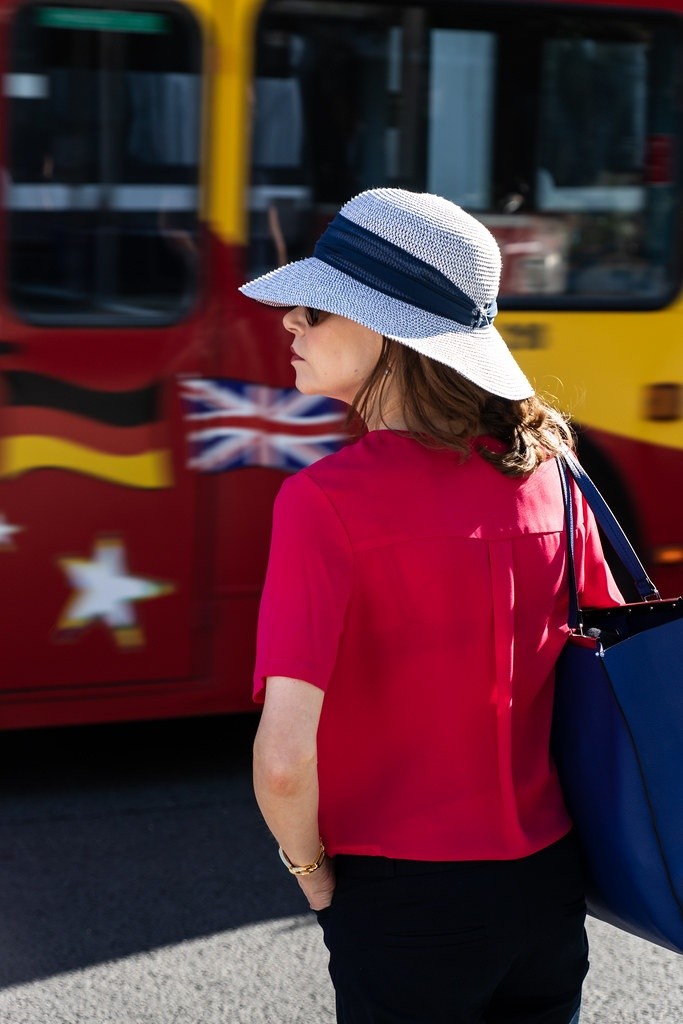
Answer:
[{"left": 541, "top": 427, "right": 683, "bottom": 955}]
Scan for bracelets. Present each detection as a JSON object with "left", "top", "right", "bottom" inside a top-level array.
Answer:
[{"left": 278, "top": 837, "right": 325, "bottom": 875}]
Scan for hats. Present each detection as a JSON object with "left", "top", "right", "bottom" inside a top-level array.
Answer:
[{"left": 238, "top": 187, "right": 535, "bottom": 401}]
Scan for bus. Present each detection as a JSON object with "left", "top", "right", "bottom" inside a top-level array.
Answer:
[{"left": 0, "top": 0, "right": 682, "bottom": 730}]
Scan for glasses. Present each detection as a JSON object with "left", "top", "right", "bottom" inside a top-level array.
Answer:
[{"left": 305, "top": 307, "right": 319, "bottom": 326}]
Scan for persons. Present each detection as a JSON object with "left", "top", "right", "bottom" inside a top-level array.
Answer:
[{"left": 236, "top": 186, "right": 626, "bottom": 1024}]
